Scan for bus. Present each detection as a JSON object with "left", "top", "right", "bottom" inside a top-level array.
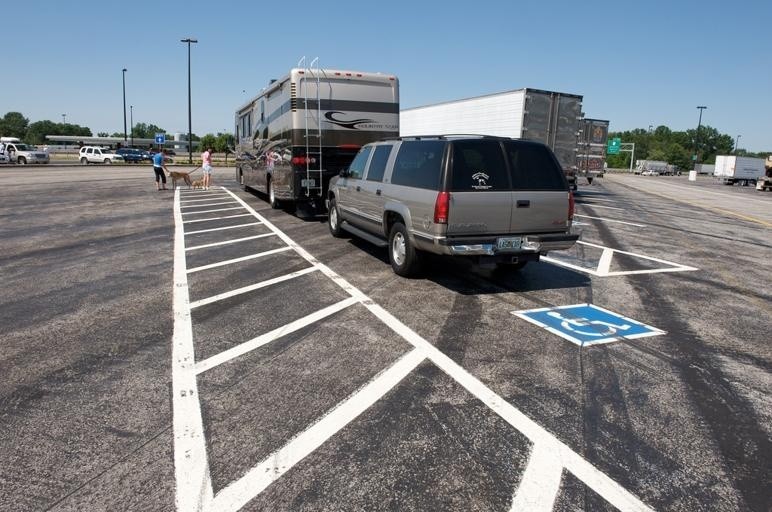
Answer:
[{"left": 234, "top": 56, "right": 400, "bottom": 217}]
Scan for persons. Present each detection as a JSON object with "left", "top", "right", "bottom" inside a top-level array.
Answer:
[
  {"left": 265, "top": 148, "right": 278, "bottom": 171},
  {"left": 43, "top": 145, "right": 48, "bottom": 152},
  {"left": 152, "top": 150, "right": 170, "bottom": 191},
  {"left": 201, "top": 147, "right": 213, "bottom": 191}
]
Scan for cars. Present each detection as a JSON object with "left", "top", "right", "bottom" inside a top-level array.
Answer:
[
  {"left": 0, "top": 137, "right": 50, "bottom": 165},
  {"left": 327, "top": 133, "right": 579, "bottom": 276},
  {"left": 642, "top": 170, "right": 659, "bottom": 176},
  {"left": 116, "top": 148, "right": 176, "bottom": 163}
]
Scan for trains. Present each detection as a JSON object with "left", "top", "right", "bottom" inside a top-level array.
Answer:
[{"left": 43, "top": 135, "right": 202, "bottom": 154}]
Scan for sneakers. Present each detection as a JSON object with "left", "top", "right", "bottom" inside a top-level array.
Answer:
[
  {"left": 157, "top": 188, "right": 168, "bottom": 190},
  {"left": 203, "top": 187, "right": 208, "bottom": 190}
]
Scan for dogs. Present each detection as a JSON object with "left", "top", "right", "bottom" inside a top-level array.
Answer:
[
  {"left": 192, "top": 177, "right": 204, "bottom": 190},
  {"left": 167, "top": 170, "right": 192, "bottom": 190}
]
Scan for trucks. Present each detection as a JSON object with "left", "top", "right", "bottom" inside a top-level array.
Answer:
[{"left": 636, "top": 159, "right": 681, "bottom": 176}]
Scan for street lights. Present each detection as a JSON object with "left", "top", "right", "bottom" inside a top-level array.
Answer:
[
  {"left": 181, "top": 38, "right": 198, "bottom": 164},
  {"left": 121, "top": 67, "right": 133, "bottom": 146},
  {"left": 61, "top": 113, "right": 67, "bottom": 153},
  {"left": 692, "top": 106, "right": 708, "bottom": 170}
]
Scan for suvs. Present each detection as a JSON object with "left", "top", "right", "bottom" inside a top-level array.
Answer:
[{"left": 79, "top": 146, "right": 125, "bottom": 164}]
